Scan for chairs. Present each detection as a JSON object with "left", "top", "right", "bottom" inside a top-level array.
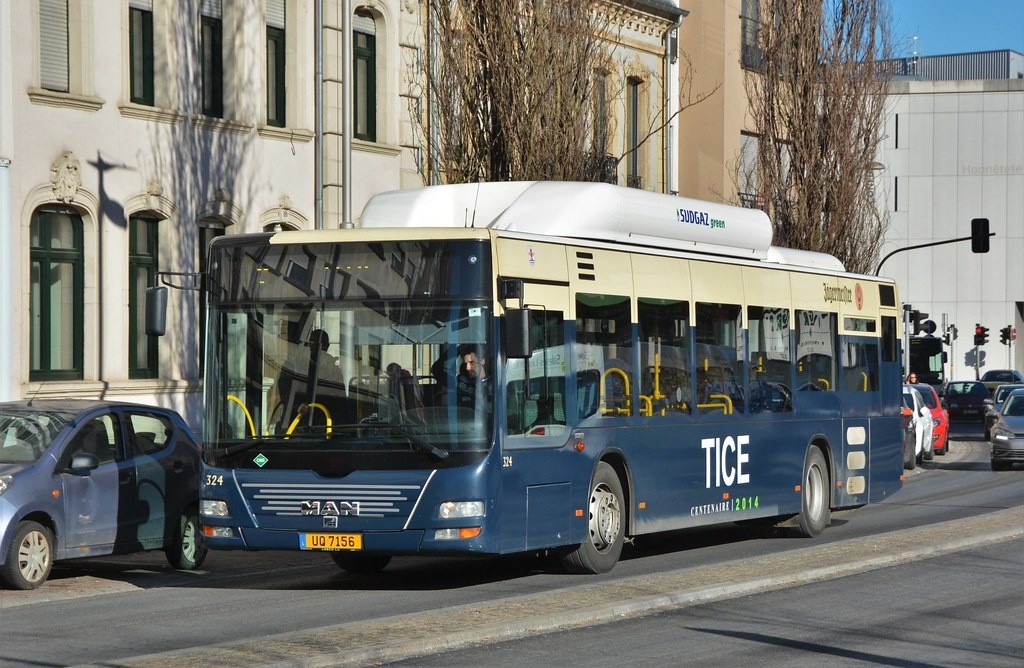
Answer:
[
  {"left": 77, "top": 420, "right": 110, "bottom": 458},
  {"left": 575, "top": 341, "right": 870, "bottom": 418}
]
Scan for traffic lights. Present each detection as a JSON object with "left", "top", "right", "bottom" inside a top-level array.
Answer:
[
  {"left": 913, "top": 309, "right": 930, "bottom": 335},
  {"left": 980, "top": 326, "right": 989, "bottom": 345},
  {"left": 1000, "top": 327, "right": 1007, "bottom": 345}
]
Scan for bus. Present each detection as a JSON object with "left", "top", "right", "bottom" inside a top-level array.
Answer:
[{"left": 143, "top": 175, "right": 908, "bottom": 577}]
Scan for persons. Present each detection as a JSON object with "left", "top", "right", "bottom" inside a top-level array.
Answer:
[
  {"left": 905, "top": 372, "right": 919, "bottom": 384},
  {"left": 387, "top": 363, "right": 411, "bottom": 379},
  {"left": 278, "top": 329, "right": 347, "bottom": 426},
  {"left": 457, "top": 346, "right": 495, "bottom": 414}
]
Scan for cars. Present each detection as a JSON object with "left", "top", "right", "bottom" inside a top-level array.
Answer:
[
  {"left": 901, "top": 384, "right": 934, "bottom": 471},
  {"left": 982, "top": 383, "right": 1024, "bottom": 440},
  {"left": 0, "top": 398, "right": 209, "bottom": 592},
  {"left": 984, "top": 388, "right": 1024, "bottom": 472},
  {"left": 907, "top": 383, "right": 951, "bottom": 455},
  {"left": 939, "top": 380, "right": 991, "bottom": 424},
  {"left": 979, "top": 368, "right": 1024, "bottom": 395}
]
